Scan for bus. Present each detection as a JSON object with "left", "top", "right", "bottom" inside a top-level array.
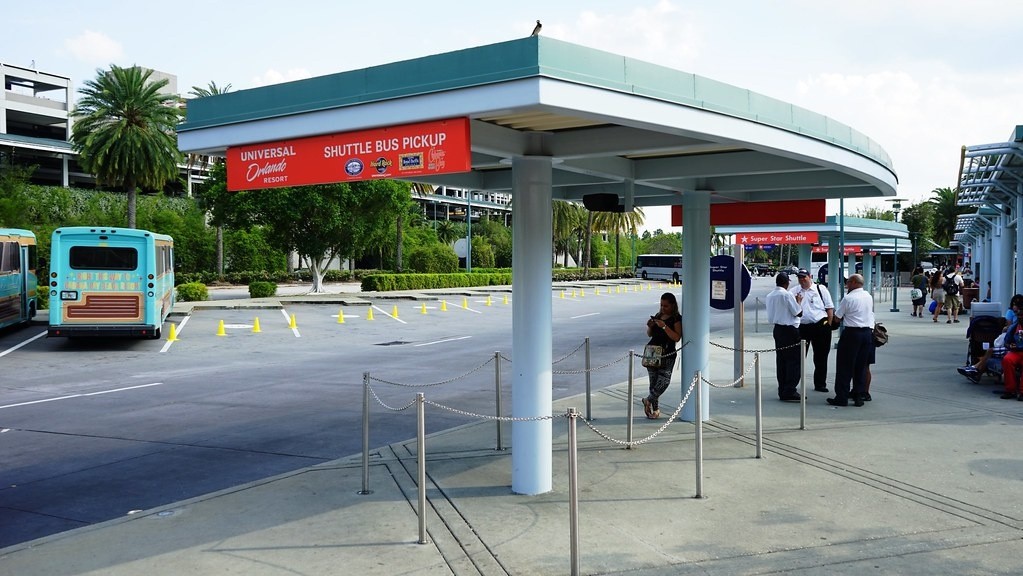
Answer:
[
  {"left": 0, "top": 228, "right": 46, "bottom": 331},
  {"left": 45, "top": 227, "right": 182, "bottom": 341},
  {"left": 634, "top": 254, "right": 682, "bottom": 283},
  {"left": 811, "top": 251, "right": 863, "bottom": 285}
]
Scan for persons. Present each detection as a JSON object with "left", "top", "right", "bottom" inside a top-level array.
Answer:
[
  {"left": 957, "top": 294, "right": 1023, "bottom": 402},
  {"left": 765, "top": 273, "right": 808, "bottom": 400},
  {"left": 752, "top": 266, "right": 758, "bottom": 280},
  {"left": 787, "top": 268, "right": 834, "bottom": 392},
  {"left": 826, "top": 274, "right": 876, "bottom": 406},
  {"left": 911, "top": 262, "right": 992, "bottom": 323},
  {"left": 642, "top": 292, "right": 682, "bottom": 419}
]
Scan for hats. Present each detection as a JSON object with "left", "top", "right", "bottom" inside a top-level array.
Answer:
[
  {"left": 797, "top": 269, "right": 809, "bottom": 276},
  {"left": 777, "top": 272, "right": 791, "bottom": 281}
]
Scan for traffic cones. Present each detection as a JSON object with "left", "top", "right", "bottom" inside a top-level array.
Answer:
[
  {"left": 486, "top": 296, "right": 491, "bottom": 306},
  {"left": 392, "top": 305, "right": 399, "bottom": 318},
  {"left": 580, "top": 288, "right": 584, "bottom": 296},
  {"left": 337, "top": 310, "right": 344, "bottom": 323},
  {"left": 367, "top": 307, "right": 375, "bottom": 321},
  {"left": 440, "top": 300, "right": 447, "bottom": 310},
  {"left": 503, "top": 295, "right": 507, "bottom": 304},
  {"left": 216, "top": 320, "right": 228, "bottom": 336},
  {"left": 166, "top": 324, "right": 180, "bottom": 341},
  {"left": 594, "top": 287, "right": 599, "bottom": 295},
  {"left": 288, "top": 314, "right": 299, "bottom": 329},
  {"left": 571, "top": 289, "right": 577, "bottom": 296},
  {"left": 560, "top": 291, "right": 560, "bottom": 298},
  {"left": 462, "top": 298, "right": 467, "bottom": 309},
  {"left": 607, "top": 281, "right": 680, "bottom": 294},
  {"left": 421, "top": 302, "right": 427, "bottom": 314},
  {"left": 251, "top": 316, "right": 263, "bottom": 333}
]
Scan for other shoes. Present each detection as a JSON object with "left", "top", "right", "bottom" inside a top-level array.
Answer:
[
  {"left": 642, "top": 398, "right": 651, "bottom": 417},
  {"left": 933, "top": 316, "right": 937, "bottom": 322},
  {"left": 1000, "top": 393, "right": 1023, "bottom": 401},
  {"left": 960, "top": 367, "right": 983, "bottom": 384},
  {"left": 849, "top": 389, "right": 871, "bottom": 401},
  {"left": 911, "top": 313, "right": 917, "bottom": 316},
  {"left": 953, "top": 320, "right": 960, "bottom": 323},
  {"left": 956, "top": 365, "right": 979, "bottom": 377},
  {"left": 827, "top": 398, "right": 848, "bottom": 406},
  {"left": 855, "top": 400, "right": 864, "bottom": 406},
  {"left": 815, "top": 386, "right": 829, "bottom": 392},
  {"left": 648, "top": 411, "right": 661, "bottom": 419},
  {"left": 779, "top": 393, "right": 807, "bottom": 400},
  {"left": 919, "top": 313, "right": 924, "bottom": 318},
  {"left": 945, "top": 319, "right": 951, "bottom": 324}
]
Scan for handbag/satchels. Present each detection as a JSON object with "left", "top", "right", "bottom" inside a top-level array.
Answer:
[
  {"left": 817, "top": 283, "right": 840, "bottom": 330},
  {"left": 642, "top": 345, "right": 664, "bottom": 368},
  {"left": 910, "top": 288, "right": 922, "bottom": 300},
  {"left": 872, "top": 323, "right": 888, "bottom": 347}
]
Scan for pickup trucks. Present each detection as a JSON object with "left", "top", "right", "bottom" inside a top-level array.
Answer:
[{"left": 749, "top": 265, "right": 777, "bottom": 277}]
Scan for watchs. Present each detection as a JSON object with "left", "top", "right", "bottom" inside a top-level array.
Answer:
[{"left": 662, "top": 324, "right": 667, "bottom": 330}]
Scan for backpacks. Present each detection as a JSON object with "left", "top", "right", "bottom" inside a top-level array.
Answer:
[{"left": 942, "top": 275, "right": 960, "bottom": 296}]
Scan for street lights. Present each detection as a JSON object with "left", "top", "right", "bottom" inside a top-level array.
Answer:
[
  {"left": 885, "top": 199, "right": 909, "bottom": 311},
  {"left": 911, "top": 232, "right": 921, "bottom": 268},
  {"left": 469, "top": 190, "right": 480, "bottom": 273}
]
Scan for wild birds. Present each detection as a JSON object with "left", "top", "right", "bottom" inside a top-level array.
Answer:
[{"left": 530, "top": 20, "right": 542, "bottom": 37}]
